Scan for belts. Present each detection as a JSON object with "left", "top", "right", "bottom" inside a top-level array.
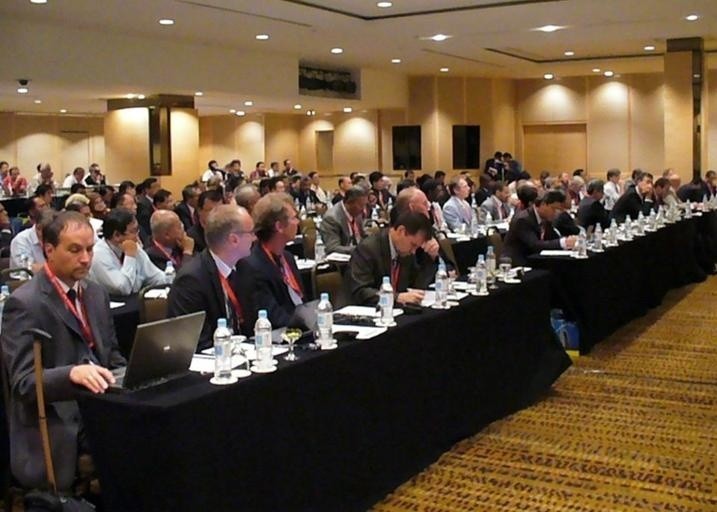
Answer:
[{"left": 278, "top": 256, "right": 303, "bottom": 306}]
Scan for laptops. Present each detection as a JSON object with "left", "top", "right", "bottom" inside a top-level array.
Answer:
[
  {"left": 249, "top": 299, "right": 320, "bottom": 344},
  {"left": 104, "top": 311, "right": 206, "bottom": 393}
]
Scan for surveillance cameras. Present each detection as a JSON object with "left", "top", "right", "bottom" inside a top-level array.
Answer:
[{"left": 19, "top": 80, "right": 28, "bottom": 85}]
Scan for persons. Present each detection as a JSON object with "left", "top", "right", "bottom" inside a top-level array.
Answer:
[
  {"left": 2, "top": 158, "right": 332, "bottom": 495},
  {"left": 476, "top": 152, "right": 717, "bottom": 267},
  {"left": 320, "top": 166, "right": 479, "bottom": 308}
]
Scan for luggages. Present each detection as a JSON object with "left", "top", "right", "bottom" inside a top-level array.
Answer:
[{"left": 19, "top": 327, "right": 104, "bottom": 511}]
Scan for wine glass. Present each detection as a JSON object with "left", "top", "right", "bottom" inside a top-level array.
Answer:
[
  {"left": 230, "top": 335, "right": 252, "bottom": 375},
  {"left": 499, "top": 257, "right": 512, "bottom": 282},
  {"left": 485, "top": 261, "right": 501, "bottom": 290},
  {"left": 467, "top": 266, "right": 481, "bottom": 290},
  {"left": 279, "top": 329, "right": 300, "bottom": 362},
  {"left": 446, "top": 276, "right": 459, "bottom": 298}
]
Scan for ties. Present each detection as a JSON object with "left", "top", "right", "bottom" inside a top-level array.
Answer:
[
  {"left": 227, "top": 271, "right": 238, "bottom": 335},
  {"left": 67, "top": 289, "right": 77, "bottom": 312}
]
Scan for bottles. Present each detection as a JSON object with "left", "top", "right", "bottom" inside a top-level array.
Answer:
[
  {"left": 452, "top": 196, "right": 494, "bottom": 237},
  {"left": 0, "top": 285, "right": 12, "bottom": 318},
  {"left": 379, "top": 276, "right": 396, "bottom": 324},
  {"left": 434, "top": 262, "right": 448, "bottom": 308},
  {"left": 212, "top": 318, "right": 232, "bottom": 379},
  {"left": 164, "top": 262, "right": 176, "bottom": 286},
  {"left": 571, "top": 193, "right": 715, "bottom": 257},
  {"left": 316, "top": 292, "right": 335, "bottom": 344},
  {"left": 254, "top": 310, "right": 273, "bottom": 363},
  {"left": 483, "top": 244, "right": 495, "bottom": 276},
  {"left": 475, "top": 254, "right": 487, "bottom": 292},
  {"left": 312, "top": 236, "right": 328, "bottom": 261}
]
